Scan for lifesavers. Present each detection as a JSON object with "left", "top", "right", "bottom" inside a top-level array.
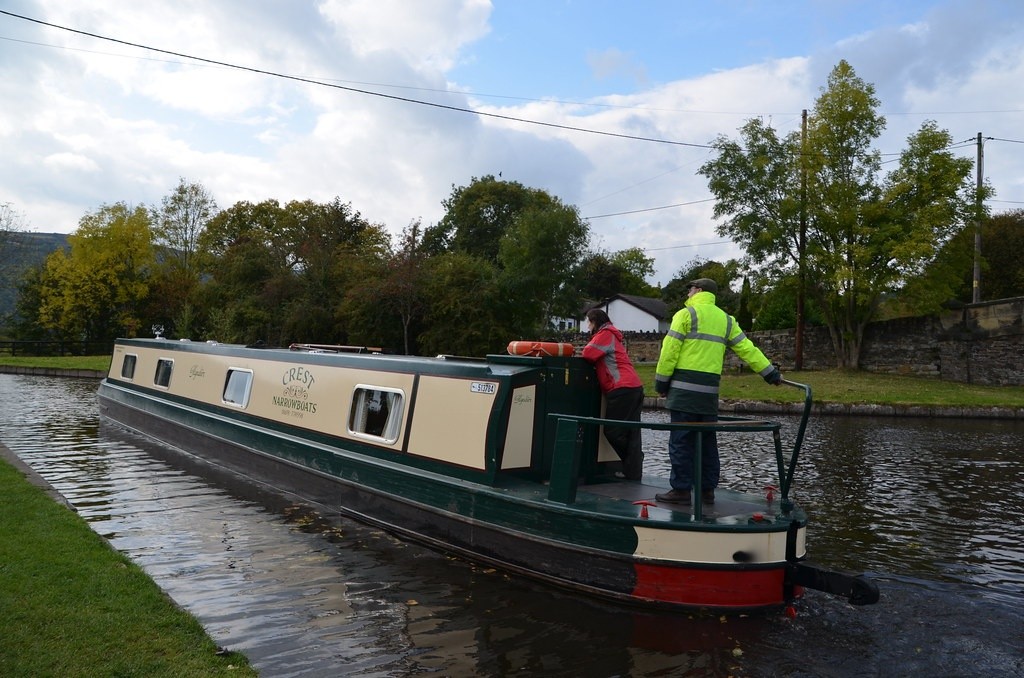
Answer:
[{"left": 507, "top": 340, "right": 573, "bottom": 357}]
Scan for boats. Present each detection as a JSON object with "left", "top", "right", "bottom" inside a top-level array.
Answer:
[{"left": 97, "top": 334, "right": 818, "bottom": 621}]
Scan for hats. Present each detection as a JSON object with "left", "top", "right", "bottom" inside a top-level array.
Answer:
[{"left": 691, "top": 278, "right": 717, "bottom": 296}]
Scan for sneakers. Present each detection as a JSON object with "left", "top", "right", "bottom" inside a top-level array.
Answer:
[
  {"left": 702, "top": 489, "right": 714, "bottom": 504},
  {"left": 655, "top": 490, "right": 691, "bottom": 505}
]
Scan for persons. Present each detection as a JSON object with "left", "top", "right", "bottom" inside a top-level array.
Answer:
[
  {"left": 582, "top": 309, "right": 644, "bottom": 480},
  {"left": 655, "top": 278, "right": 783, "bottom": 504}
]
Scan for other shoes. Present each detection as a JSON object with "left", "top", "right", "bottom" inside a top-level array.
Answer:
[
  {"left": 641, "top": 452, "right": 644, "bottom": 462},
  {"left": 615, "top": 471, "right": 641, "bottom": 480}
]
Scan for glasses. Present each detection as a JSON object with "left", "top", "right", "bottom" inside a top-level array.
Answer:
[{"left": 689, "top": 288, "right": 703, "bottom": 293}]
